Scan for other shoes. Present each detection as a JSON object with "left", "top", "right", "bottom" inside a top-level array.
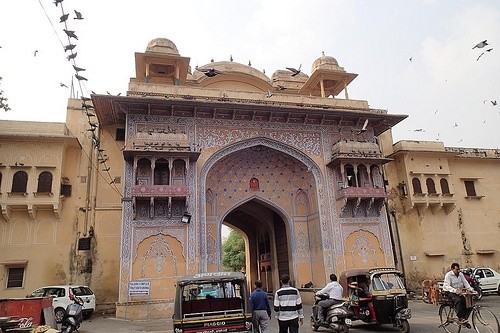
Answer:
[
  {"left": 315, "top": 320, "right": 323, "bottom": 325},
  {"left": 459, "top": 318, "right": 468, "bottom": 323},
  {"left": 463, "top": 323, "right": 471, "bottom": 329}
]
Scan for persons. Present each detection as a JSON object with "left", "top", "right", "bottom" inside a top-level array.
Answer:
[
  {"left": 464, "top": 269, "right": 475, "bottom": 285},
  {"left": 248, "top": 280, "right": 272, "bottom": 333},
  {"left": 273, "top": 274, "right": 305, "bottom": 333},
  {"left": 315, "top": 274, "right": 344, "bottom": 320},
  {"left": 443, "top": 263, "right": 477, "bottom": 328},
  {"left": 358, "top": 278, "right": 376, "bottom": 321}
]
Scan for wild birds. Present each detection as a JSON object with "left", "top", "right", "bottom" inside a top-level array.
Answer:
[
  {"left": 121, "top": 141, "right": 203, "bottom": 151},
  {"left": 490, "top": 99, "right": 497, "bottom": 105},
  {"left": 14, "top": 161, "right": 24, "bottom": 166},
  {"left": 117, "top": 92, "right": 121, "bottom": 96},
  {"left": 346, "top": 150, "right": 382, "bottom": 157},
  {"left": 34, "top": 50, "right": 39, "bottom": 56},
  {"left": 331, "top": 118, "right": 379, "bottom": 146},
  {"left": 137, "top": 128, "right": 179, "bottom": 135},
  {"left": 144, "top": 115, "right": 149, "bottom": 122},
  {"left": 203, "top": 68, "right": 221, "bottom": 78},
  {"left": 483, "top": 121, "right": 485, "bottom": 123},
  {"left": 265, "top": 63, "right": 303, "bottom": 99},
  {"left": 434, "top": 109, "right": 438, "bottom": 114},
  {"left": 409, "top": 56, "right": 413, "bottom": 61},
  {"left": 415, "top": 128, "right": 422, "bottom": 131},
  {"left": 51, "top": 0, "right": 118, "bottom": 187},
  {"left": 455, "top": 123, "right": 458, "bottom": 127},
  {"left": 0, "top": 162, "right": 2, "bottom": 165},
  {"left": 471, "top": 39, "right": 494, "bottom": 61},
  {"left": 374, "top": 184, "right": 379, "bottom": 189}
]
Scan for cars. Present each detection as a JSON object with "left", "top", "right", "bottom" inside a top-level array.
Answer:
[{"left": 460, "top": 267, "right": 500, "bottom": 296}]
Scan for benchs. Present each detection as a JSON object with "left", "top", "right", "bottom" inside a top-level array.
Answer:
[{"left": 182, "top": 297, "right": 241, "bottom": 314}]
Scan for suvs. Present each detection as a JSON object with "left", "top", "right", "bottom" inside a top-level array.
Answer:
[{"left": 25, "top": 284, "right": 96, "bottom": 323}]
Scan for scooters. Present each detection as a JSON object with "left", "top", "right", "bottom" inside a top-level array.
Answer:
[{"left": 463, "top": 275, "right": 483, "bottom": 301}]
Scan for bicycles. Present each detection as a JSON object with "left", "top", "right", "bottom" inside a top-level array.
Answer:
[{"left": 437, "top": 291, "right": 500, "bottom": 333}]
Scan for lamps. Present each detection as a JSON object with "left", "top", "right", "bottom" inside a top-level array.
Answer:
[{"left": 181, "top": 210, "right": 191, "bottom": 224}]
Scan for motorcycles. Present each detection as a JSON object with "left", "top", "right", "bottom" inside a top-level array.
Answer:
[
  {"left": 171, "top": 271, "right": 254, "bottom": 333},
  {"left": 61, "top": 294, "right": 85, "bottom": 333},
  {"left": 310, "top": 289, "right": 354, "bottom": 333},
  {"left": 338, "top": 266, "right": 412, "bottom": 333}
]
